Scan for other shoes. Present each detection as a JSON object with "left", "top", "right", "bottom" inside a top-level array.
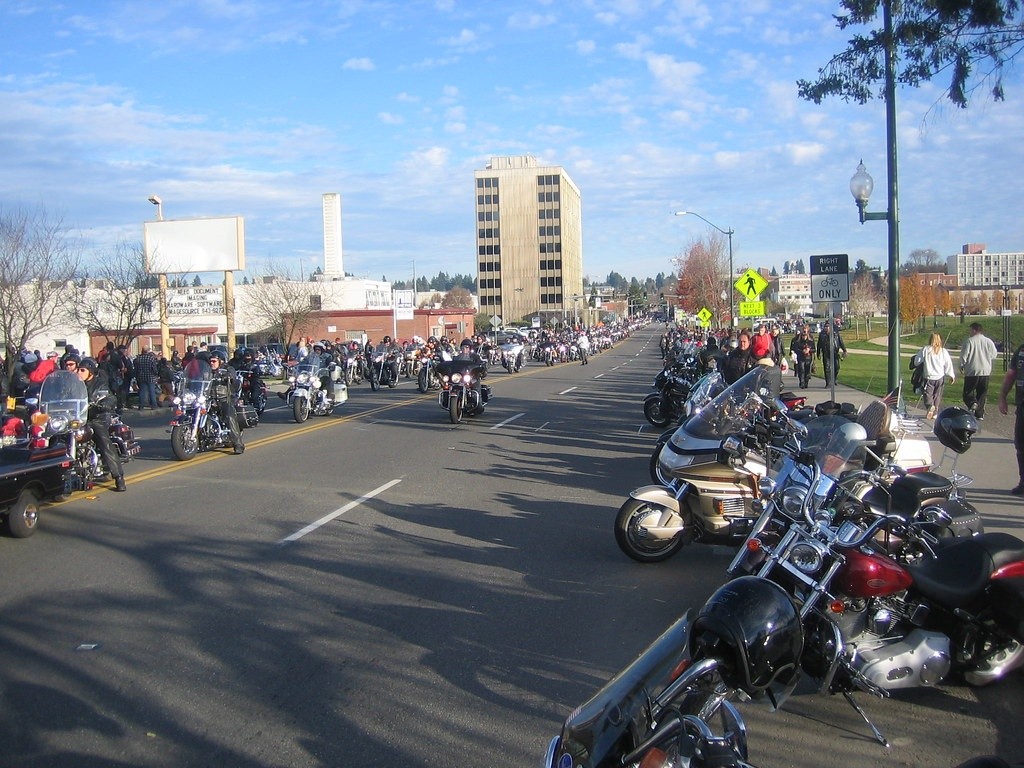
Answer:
[
  {"left": 234, "top": 445, "right": 244, "bottom": 454},
  {"left": 802, "top": 385, "right": 808, "bottom": 389},
  {"left": 927, "top": 406, "right": 935, "bottom": 419},
  {"left": 277, "top": 391, "right": 286, "bottom": 399},
  {"left": 1011, "top": 484, "right": 1024, "bottom": 495},
  {"left": 976, "top": 417, "right": 983, "bottom": 420},
  {"left": 116, "top": 404, "right": 158, "bottom": 415},
  {"left": 932, "top": 415, "right": 937, "bottom": 419},
  {"left": 970, "top": 403, "right": 977, "bottom": 414},
  {"left": 835, "top": 382, "right": 837, "bottom": 386},
  {"left": 825, "top": 385, "right": 830, "bottom": 389}
]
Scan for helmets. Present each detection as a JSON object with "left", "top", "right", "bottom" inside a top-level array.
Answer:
[
  {"left": 64, "top": 354, "right": 81, "bottom": 367},
  {"left": 689, "top": 575, "right": 804, "bottom": 690},
  {"left": 76, "top": 359, "right": 96, "bottom": 373},
  {"left": 193, "top": 329, "right": 558, "bottom": 369},
  {"left": 46, "top": 351, "right": 58, "bottom": 357},
  {"left": 934, "top": 406, "right": 977, "bottom": 454}
]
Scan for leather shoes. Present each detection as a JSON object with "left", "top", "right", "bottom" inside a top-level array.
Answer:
[{"left": 115, "top": 475, "right": 126, "bottom": 491}]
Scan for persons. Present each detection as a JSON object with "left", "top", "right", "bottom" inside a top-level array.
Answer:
[
  {"left": 959, "top": 322, "right": 997, "bottom": 420},
  {"left": 817, "top": 322, "right": 847, "bottom": 389},
  {"left": 208, "top": 348, "right": 261, "bottom": 453},
  {"left": 708, "top": 328, "right": 750, "bottom": 352},
  {"left": 790, "top": 323, "right": 816, "bottom": 388},
  {"left": 278, "top": 335, "right": 522, "bottom": 411},
  {"left": 768, "top": 325, "right": 786, "bottom": 367},
  {"left": 695, "top": 337, "right": 727, "bottom": 380},
  {"left": 750, "top": 324, "right": 772, "bottom": 360},
  {"left": 576, "top": 333, "right": 589, "bottom": 365},
  {"left": 64, "top": 355, "right": 126, "bottom": 491},
  {"left": 721, "top": 333, "right": 759, "bottom": 386},
  {"left": 915, "top": 332, "right": 956, "bottom": 419},
  {"left": 535, "top": 326, "right": 630, "bottom": 360},
  {"left": 97, "top": 341, "right": 210, "bottom": 410},
  {"left": 998, "top": 343, "right": 1024, "bottom": 496},
  {"left": 4, "top": 344, "right": 80, "bottom": 396},
  {"left": 659, "top": 332, "right": 670, "bottom": 358}
]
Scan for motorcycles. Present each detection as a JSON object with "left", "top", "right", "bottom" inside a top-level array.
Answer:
[
  {"left": 430, "top": 350, "right": 493, "bottom": 425},
  {"left": 539, "top": 603, "right": 765, "bottom": 768},
  {"left": 0, "top": 304, "right": 984, "bottom": 570},
  {"left": 713, "top": 410, "right": 1024, "bottom": 752},
  {"left": 166, "top": 358, "right": 260, "bottom": 461},
  {"left": 281, "top": 352, "right": 348, "bottom": 423}
]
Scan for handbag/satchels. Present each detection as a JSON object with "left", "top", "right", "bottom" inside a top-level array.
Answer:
[
  {"left": 790, "top": 351, "right": 798, "bottom": 365},
  {"left": 811, "top": 360, "right": 817, "bottom": 374},
  {"left": 780, "top": 358, "right": 789, "bottom": 375}
]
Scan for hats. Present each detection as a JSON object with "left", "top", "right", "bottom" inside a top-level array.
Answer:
[{"left": 118, "top": 345, "right": 127, "bottom": 349}]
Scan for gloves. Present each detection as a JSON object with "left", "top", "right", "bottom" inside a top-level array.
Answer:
[{"left": 98, "top": 401, "right": 112, "bottom": 412}]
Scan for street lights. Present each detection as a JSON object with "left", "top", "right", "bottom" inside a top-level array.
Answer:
[
  {"left": 850, "top": 0, "right": 905, "bottom": 419},
  {"left": 146, "top": 195, "right": 162, "bottom": 224},
  {"left": 674, "top": 211, "right": 734, "bottom": 335}
]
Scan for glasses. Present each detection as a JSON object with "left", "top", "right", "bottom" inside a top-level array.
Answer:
[
  {"left": 77, "top": 369, "right": 87, "bottom": 372},
  {"left": 65, "top": 363, "right": 76, "bottom": 366}
]
